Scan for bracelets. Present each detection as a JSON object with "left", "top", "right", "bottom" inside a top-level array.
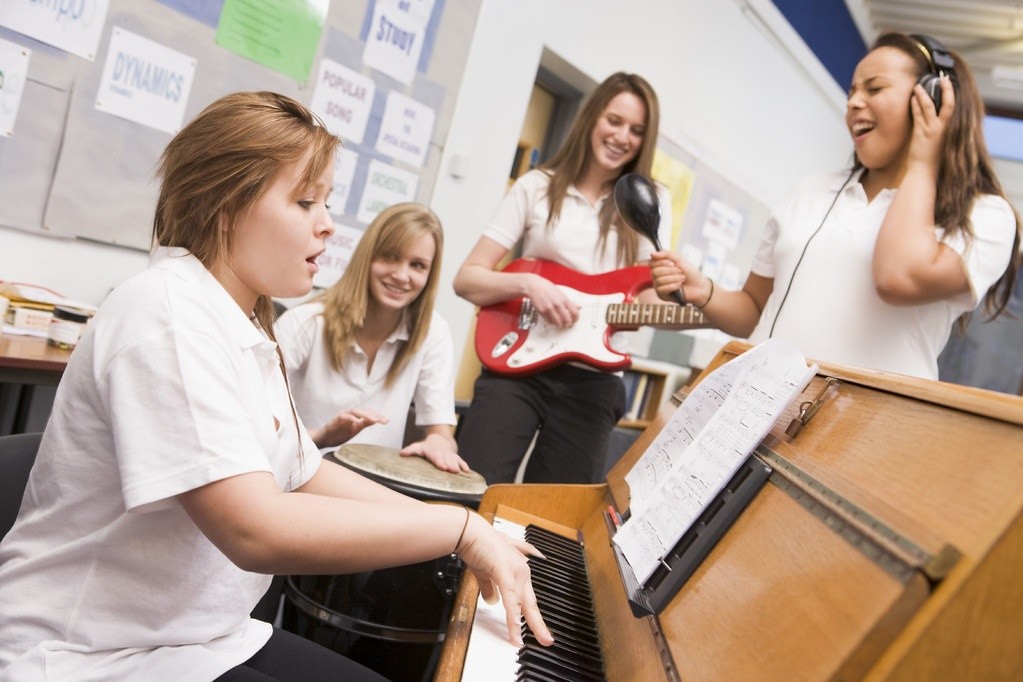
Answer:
[
  {"left": 451, "top": 506, "right": 469, "bottom": 560},
  {"left": 694, "top": 276, "right": 714, "bottom": 309}
]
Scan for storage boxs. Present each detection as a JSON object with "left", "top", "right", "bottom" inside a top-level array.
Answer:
[{"left": 4, "top": 308, "right": 54, "bottom": 329}]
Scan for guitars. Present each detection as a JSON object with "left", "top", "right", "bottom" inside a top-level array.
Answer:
[{"left": 475, "top": 255, "right": 716, "bottom": 375}]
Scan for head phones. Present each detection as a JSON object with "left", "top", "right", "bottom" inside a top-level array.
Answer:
[{"left": 857, "top": 34, "right": 955, "bottom": 171}]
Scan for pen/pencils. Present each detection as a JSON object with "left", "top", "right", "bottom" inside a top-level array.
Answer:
[{"left": 607, "top": 504, "right": 624, "bottom": 533}]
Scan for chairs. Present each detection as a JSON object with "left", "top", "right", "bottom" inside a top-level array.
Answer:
[{"left": 0, "top": 433, "right": 44, "bottom": 541}]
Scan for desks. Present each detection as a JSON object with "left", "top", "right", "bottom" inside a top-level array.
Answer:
[{"left": 0, "top": 331, "right": 76, "bottom": 435}]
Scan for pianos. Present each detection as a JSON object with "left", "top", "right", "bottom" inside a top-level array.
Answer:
[{"left": 417, "top": 340, "right": 1023, "bottom": 682}]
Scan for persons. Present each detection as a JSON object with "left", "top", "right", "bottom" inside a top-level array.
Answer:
[
  {"left": 276, "top": 203, "right": 470, "bottom": 474},
  {"left": 454, "top": 72, "right": 671, "bottom": 487},
  {"left": 0, "top": 92, "right": 556, "bottom": 682},
  {"left": 658, "top": 32, "right": 1023, "bottom": 379}
]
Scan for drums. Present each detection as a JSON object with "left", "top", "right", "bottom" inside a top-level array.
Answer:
[{"left": 281, "top": 442, "right": 488, "bottom": 682}]
